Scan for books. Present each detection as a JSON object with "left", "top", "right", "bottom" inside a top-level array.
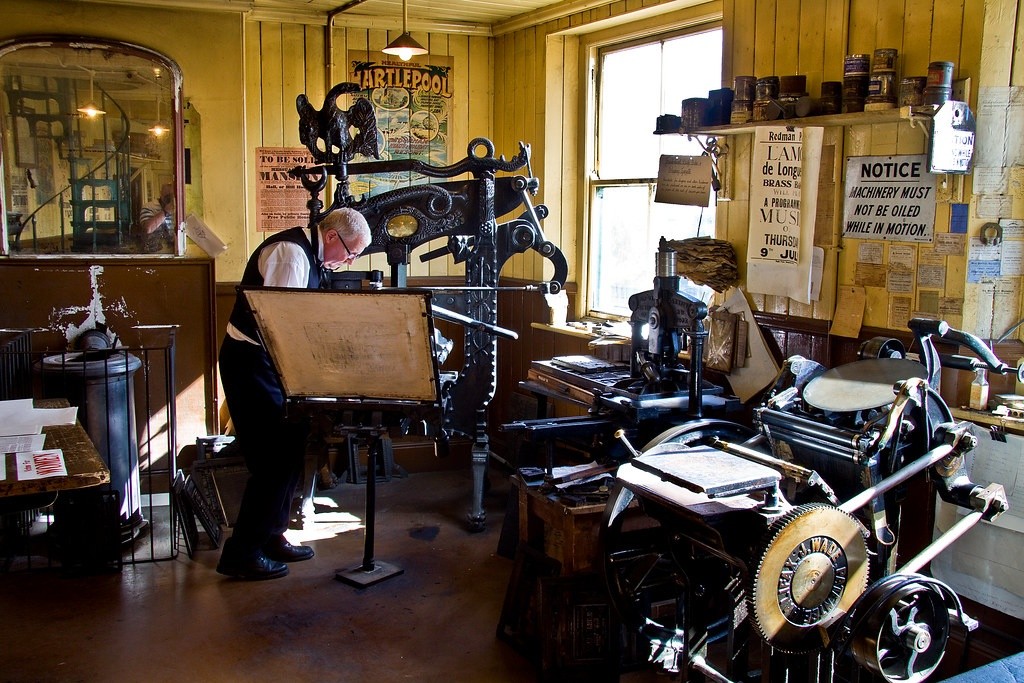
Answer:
[{"left": 552, "top": 354, "right": 629, "bottom": 375}]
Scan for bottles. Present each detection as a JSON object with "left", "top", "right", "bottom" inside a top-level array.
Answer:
[{"left": 971, "top": 368, "right": 989, "bottom": 409}]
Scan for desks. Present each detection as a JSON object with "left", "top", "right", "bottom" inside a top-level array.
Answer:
[
  {"left": 0, "top": 399, "right": 110, "bottom": 497},
  {"left": 508, "top": 473, "right": 796, "bottom": 577}
]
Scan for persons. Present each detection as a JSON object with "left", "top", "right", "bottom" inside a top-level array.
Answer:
[
  {"left": 215, "top": 207, "right": 372, "bottom": 580},
  {"left": 140, "top": 184, "right": 176, "bottom": 254},
  {"left": 982, "top": 228, "right": 997, "bottom": 246}
]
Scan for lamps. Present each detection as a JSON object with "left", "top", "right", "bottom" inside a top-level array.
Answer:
[
  {"left": 382, "top": 0, "right": 429, "bottom": 62},
  {"left": 76, "top": 76, "right": 106, "bottom": 116},
  {"left": 149, "top": 104, "right": 170, "bottom": 136}
]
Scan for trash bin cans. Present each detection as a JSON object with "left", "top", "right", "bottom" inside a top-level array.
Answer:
[{"left": 40, "top": 349, "right": 150, "bottom": 548}]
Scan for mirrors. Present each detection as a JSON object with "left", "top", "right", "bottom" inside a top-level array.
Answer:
[{"left": 0, "top": 31, "right": 187, "bottom": 259}]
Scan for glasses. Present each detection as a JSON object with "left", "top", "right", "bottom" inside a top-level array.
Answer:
[{"left": 329, "top": 228, "right": 362, "bottom": 260}]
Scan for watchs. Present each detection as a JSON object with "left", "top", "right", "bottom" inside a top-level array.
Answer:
[{"left": 163, "top": 210, "right": 169, "bottom": 217}]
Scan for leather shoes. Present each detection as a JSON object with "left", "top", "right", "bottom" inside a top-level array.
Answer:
[
  {"left": 266, "top": 543, "right": 315, "bottom": 563},
  {"left": 216, "top": 549, "right": 288, "bottom": 580}
]
